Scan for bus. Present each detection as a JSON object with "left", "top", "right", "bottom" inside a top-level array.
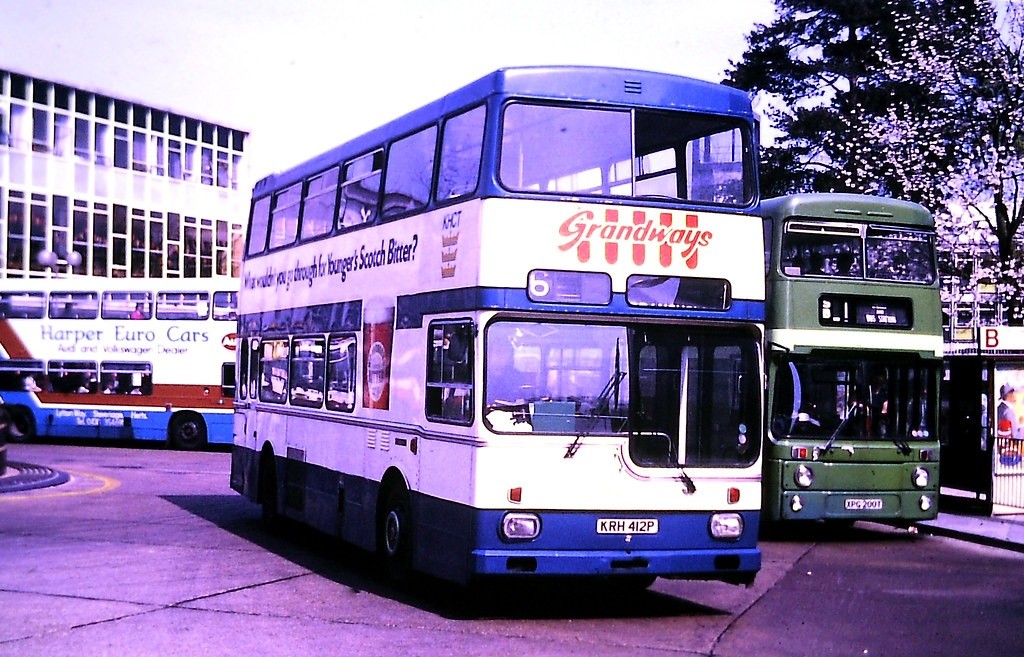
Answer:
[
  {"left": 0, "top": 276, "right": 243, "bottom": 451},
  {"left": 532, "top": 188, "right": 945, "bottom": 529},
  {"left": 223, "top": 60, "right": 770, "bottom": 607}
]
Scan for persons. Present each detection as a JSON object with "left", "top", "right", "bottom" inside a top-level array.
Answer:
[
  {"left": 485, "top": 340, "right": 539, "bottom": 407},
  {"left": 18, "top": 370, "right": 153, "bottom": 396},
  {"left": 997, "top": 381, "right": 1024, "bottom": 466}
]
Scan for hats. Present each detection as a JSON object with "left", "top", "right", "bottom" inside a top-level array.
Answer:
[{"left": 998, "top": 383, "right": 1013, "bottom": 400}]
